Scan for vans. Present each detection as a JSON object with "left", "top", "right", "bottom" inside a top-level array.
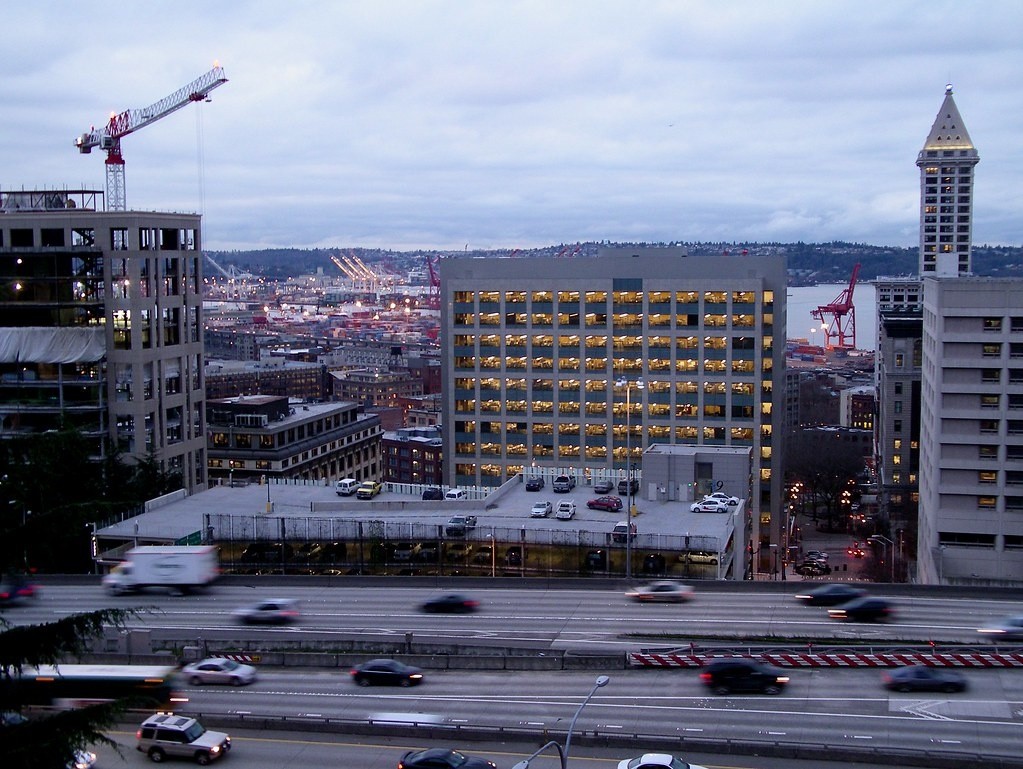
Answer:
[
  {"left": 618, "top": 478, "right": 639, "bottom": 496},
  {"left": 612, "top": 520, "right": 638, "bottom": 544},
  {"left": 335, "top": 478, "right": 362, "bottom": 496}
]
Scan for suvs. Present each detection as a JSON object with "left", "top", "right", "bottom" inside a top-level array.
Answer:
[
  {"left": 136, "top": 711, "right": 232, "bottom": 766},
  {"left": 701, "top": 658, "right": 791, "bottom": 698},
  {"left": 586, "top": 495, "right": 623, "bottom": 512},
  {"left": 552, "top": 474, "right": 577, "bottom": 493},
  {"left": 525, "top": 476, "right": 545, "bottom": 492}
]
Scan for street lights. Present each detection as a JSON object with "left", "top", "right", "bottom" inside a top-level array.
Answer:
[
  {"left": 510, "top": 675, "right": 610, "bottom": 769},
  {"left": 871, "top": 534, "right": 895, "bottom": 583},
  {"left": 867, "top": 537, "right": 887, "bottom": 581}
]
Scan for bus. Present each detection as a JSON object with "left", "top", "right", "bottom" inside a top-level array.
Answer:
[{"left": 0, "top": 663, "right": 179, "bottom": 717}]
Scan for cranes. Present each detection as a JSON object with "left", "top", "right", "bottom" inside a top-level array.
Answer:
[{"left": 72, "top": 58, "right": 231, "bottom": 299}]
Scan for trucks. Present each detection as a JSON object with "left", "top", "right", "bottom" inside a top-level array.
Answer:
[{"left": 100, "top": 545, "right": 220, "bottom": 597}]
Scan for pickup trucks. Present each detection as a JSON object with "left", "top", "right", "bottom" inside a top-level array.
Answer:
[
  {"left": 445, "top": 514, "right": 478, "bottom": 537},
  {"left": 355, "top": 480, "right": 382, "bottom": 500},
  {"left": 555, "top": 502, "right": 577, "bottom": 520}
]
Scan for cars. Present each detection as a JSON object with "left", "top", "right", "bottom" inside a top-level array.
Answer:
[
  {"left": 880, "top": 663, "right": 967, "bottom": 694},
  {"left": 221, "top": 540, "right": 528, "bottom": 578},
  {"left": 395, "top": 746, "right": 498, "bottom": 769},
  {"left": 231, "top": 598, "right": 296, "bottom": 625},
  {"left": 0, "top": 709, "right": 29, "bottom": 727},
  {"left": 616, "top": 753, "right": 709, "bottom": 769},
  {"left": 978, "top": 617, "right": 1023, "bottom": 643},
  {"left": 0, "top": 573, "right": 33, "bottom": 606},
  {"left": 351, "top": 658, "right": 424, "bottom": 688},
  {"left": 583, "top": 547, "right": 667, "bottom": 575},
  {"left": 678, "top": 551, "right": 718, "bottom": 565},
  {"left": 702, "top": 491, "right": 741, "bottom": 506},
  {"left": 629, "top": 580, "right": 697, "bottom": 603},
  {"left": 421, "top": 592, "right": 480, "bottom": 616},
  {"left": 422, "top": 487, "right": 443, "bottom": 500},
  {"left": 690, "top": 499, "right": 729, "bottom": 513},
  {"left": 793, "top": 548, "right": 831, "bottom": 576},
  {"left": 593, "top": 480, "right": 614, "bottom": 494},
  {"left": 793, "top": 582, "right": 895, "bottom": 623},
  {"left": 180, "top": 657, "right": 259, "bottom": 688},
  {"left": 65, "top": 748, "right": 98, "bottom": 769},
  {"left": 444, "top": 488, "right": 467, "bottom": 500},
  {"left": 530, "top": 500, "right": 553, "bottom": 518}
]
